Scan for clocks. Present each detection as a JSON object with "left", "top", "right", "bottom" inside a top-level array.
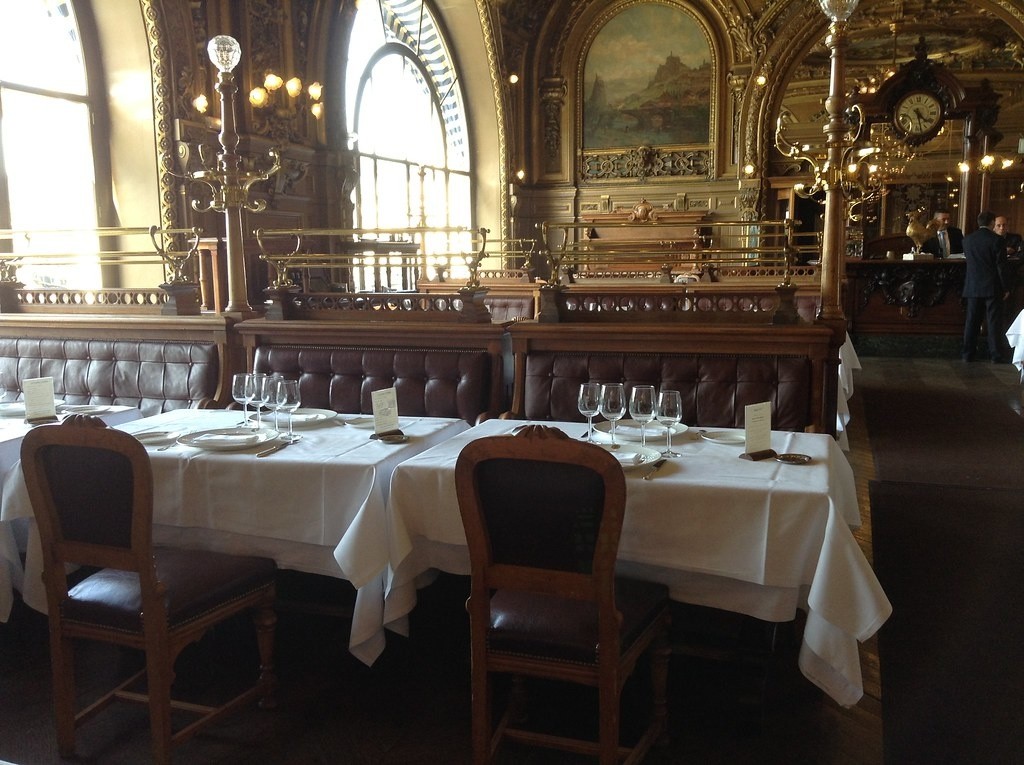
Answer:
[{"left": 893, "top": 89, "right": 943, "bottom": 139}]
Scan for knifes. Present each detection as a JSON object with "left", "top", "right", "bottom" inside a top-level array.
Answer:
[
  {"left": 643, "top": 458, "right": 666, "bottom": 479},
  {"left": 256, "top": 439, "right": 298, "bottom": 457},
  {"left": 582, "top": 426, "right": 593, "bottom": 437}
]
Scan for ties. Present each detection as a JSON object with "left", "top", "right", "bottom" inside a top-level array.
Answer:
[{"left": 939, "top": 232, "right": 947, "bottom": 258}]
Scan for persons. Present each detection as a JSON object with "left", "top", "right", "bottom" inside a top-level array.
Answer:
[
  {"left": 960, "top": 210, "right": 1012, "bottom": 365},
  {"left": 992, "top": 216, "right": 1023, "bottom": 258},
  {"left": 919, "top": 209, "right": 966, "bottom": 259}
]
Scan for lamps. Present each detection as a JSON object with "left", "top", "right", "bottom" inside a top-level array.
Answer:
[{"left": 183, "top": 68, "right": 324, "bottom": 120}]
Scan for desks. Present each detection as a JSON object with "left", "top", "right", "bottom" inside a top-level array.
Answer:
[
  {"left": 384, "top": 418, "right": 894, "bottom": 734},
  {"left": 0, "top": 401, "right": 146, "bottom": 625},
  {"left": 103, "top": 408, "right": 473, "bottom": 689}
]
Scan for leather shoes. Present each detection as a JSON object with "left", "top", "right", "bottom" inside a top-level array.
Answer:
[{"left": 991, "top": 352, "right": 1004, "bottom": 364}]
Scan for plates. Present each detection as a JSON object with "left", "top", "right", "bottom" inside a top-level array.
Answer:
[
  {"left": 701, "top": 429, "right": 747, "bottom": 444},
  {"left": 775, "top": 452, "right": 811, "bottom": 464},
  {"left": 67, "top": 405, "right": 111, "bottom": 415},
  {"left": 594, "top": 420, "right": 688, "bottom": 442},
  {"left": 176, "top": 427, "right": 280, "bottom": 451},
  {"left": 250, "top": 408, "right": 338, "bottom": 427},
  {"left": 587, "top": 442, "right": 661, "bottom": 467}
]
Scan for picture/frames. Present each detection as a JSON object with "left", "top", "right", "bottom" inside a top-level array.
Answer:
[{"left": 576, "top": 0, "right": 716, "bottom": 155}]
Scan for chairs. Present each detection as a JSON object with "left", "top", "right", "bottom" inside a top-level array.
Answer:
[
  {"left": 453, "top": 422, "right": 676, "bottom": 765},
  {"left": 19, "top": 414, "right": 282, "bottom": 765}
]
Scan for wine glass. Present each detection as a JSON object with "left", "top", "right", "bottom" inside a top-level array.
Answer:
[
  {"left": 578, "top": 382, "right": 683, "bottom": 459},
  {"left": 231, "top": 371, "right": 303, "bottom": 446}
]
